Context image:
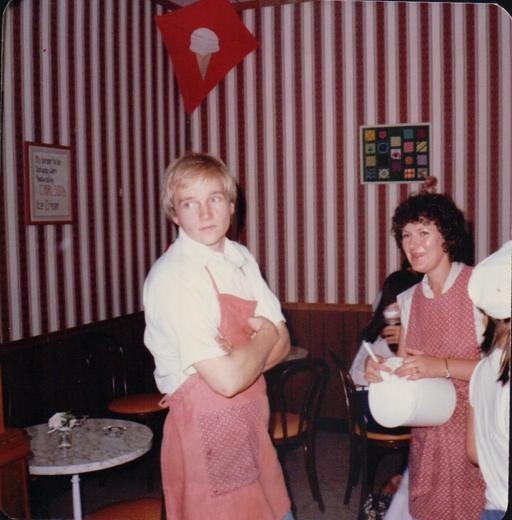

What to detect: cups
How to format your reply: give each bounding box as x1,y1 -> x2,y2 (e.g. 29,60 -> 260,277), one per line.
383,310 -> 401,352
55,431 -> 72,450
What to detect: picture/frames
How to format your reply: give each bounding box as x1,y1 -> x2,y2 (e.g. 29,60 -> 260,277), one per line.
358,122 -> 432,186
23,140 -> 77,227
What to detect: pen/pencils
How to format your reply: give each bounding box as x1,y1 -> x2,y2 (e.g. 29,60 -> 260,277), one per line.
361,340 -> 380,363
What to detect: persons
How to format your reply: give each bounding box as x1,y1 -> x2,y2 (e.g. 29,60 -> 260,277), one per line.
142,151 -> 294,519
347,194 -> 511,520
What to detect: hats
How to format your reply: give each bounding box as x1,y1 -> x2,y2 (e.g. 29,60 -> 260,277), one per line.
467,239 -> 512,320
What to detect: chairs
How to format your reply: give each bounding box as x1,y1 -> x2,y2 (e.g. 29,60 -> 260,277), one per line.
269,361 -> 412,518
84,348 -> 165,427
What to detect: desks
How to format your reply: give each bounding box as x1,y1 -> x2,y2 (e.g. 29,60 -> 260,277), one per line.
19,418 -> 156,520
